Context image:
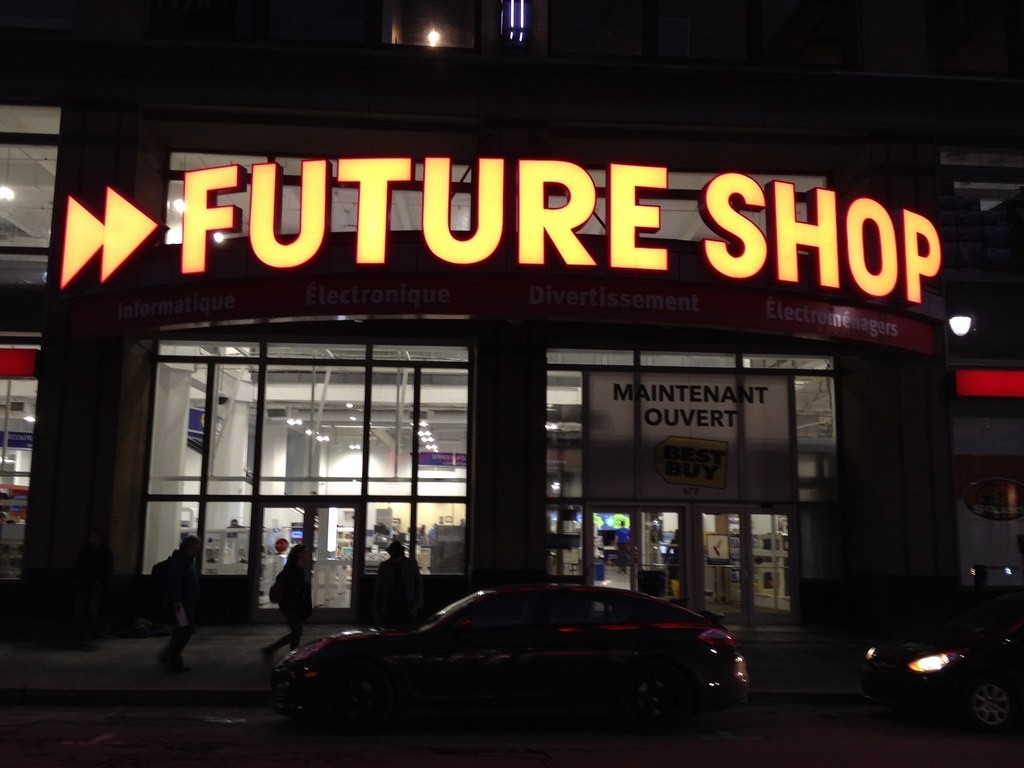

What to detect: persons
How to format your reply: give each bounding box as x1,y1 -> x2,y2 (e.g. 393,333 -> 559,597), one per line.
615,519 -> 630,573
161,536 -> 200,663
378,540 -> 424,626
71,528 -> 115,640
260,545 -> 310,657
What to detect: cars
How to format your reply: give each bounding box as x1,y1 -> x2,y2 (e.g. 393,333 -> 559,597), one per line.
270,584 -> 750,739
858,589 -> 1024,736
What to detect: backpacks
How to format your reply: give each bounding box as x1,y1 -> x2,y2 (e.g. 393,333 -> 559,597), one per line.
146,560 -> 178,603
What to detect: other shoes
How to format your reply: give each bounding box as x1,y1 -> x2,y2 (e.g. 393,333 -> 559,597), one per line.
74,632 -> 91,641
162,654 -> 183,669
262,648 -> 275,665
